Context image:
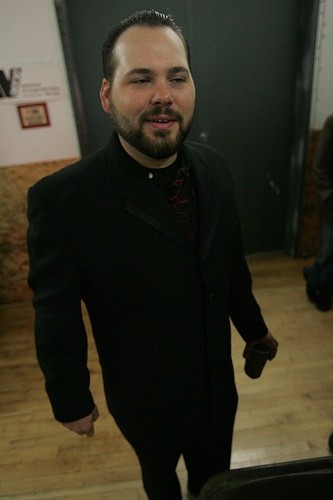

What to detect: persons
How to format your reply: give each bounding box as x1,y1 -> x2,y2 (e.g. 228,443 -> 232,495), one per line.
27,9 -> 279,498
